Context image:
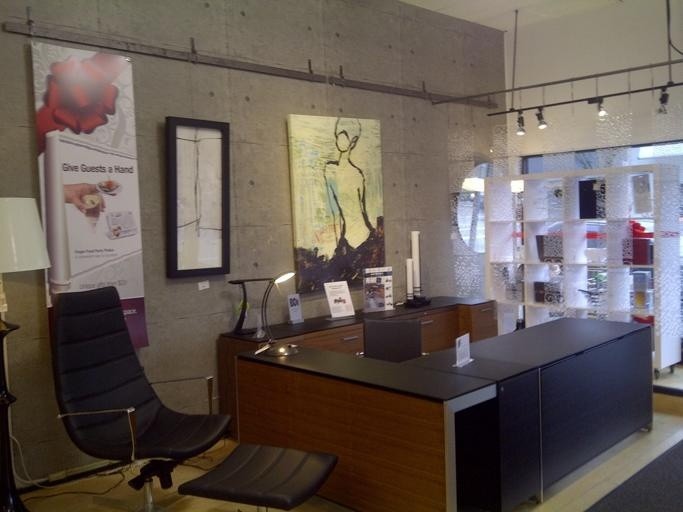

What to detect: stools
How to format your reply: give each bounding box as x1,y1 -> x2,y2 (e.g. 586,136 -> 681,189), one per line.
179,441 -> 339,512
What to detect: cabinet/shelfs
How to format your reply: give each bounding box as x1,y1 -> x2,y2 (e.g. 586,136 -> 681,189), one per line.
483,164 -> 681,378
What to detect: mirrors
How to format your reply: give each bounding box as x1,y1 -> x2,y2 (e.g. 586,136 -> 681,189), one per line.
457,162 -> 486,254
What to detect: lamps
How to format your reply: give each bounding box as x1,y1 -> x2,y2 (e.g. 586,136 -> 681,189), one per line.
596,100 -> 607,117
656,88 -> 670,115
535,107 -> 549,131
0,196 -> 53,493
260,271 -> 299,356
514,110 -> 528,137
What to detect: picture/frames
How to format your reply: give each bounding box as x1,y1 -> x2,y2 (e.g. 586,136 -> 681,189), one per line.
164,115 -> 231,278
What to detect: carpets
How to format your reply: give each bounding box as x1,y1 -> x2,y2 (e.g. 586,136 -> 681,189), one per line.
584,438 -> 682,511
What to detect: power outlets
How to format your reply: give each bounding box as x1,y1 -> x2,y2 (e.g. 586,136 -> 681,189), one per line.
197,280 -> 210,292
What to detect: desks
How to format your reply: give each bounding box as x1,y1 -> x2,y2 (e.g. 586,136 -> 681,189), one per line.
217,296 -> 499,421
237,341 -> 497,512
411,317 -> 655,512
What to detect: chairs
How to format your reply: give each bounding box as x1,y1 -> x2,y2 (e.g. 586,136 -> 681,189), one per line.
50,286 -> 232,512
